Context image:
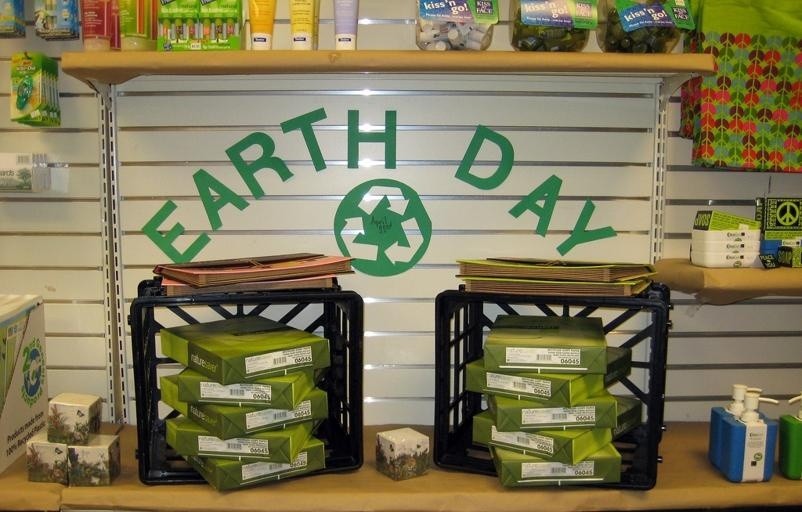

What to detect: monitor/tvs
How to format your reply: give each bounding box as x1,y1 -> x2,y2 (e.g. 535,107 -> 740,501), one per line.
457,256 -> 656,296
153,253 -> 355,297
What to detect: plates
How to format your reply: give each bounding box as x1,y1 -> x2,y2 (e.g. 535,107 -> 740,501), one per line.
57,48 -> 798,512
